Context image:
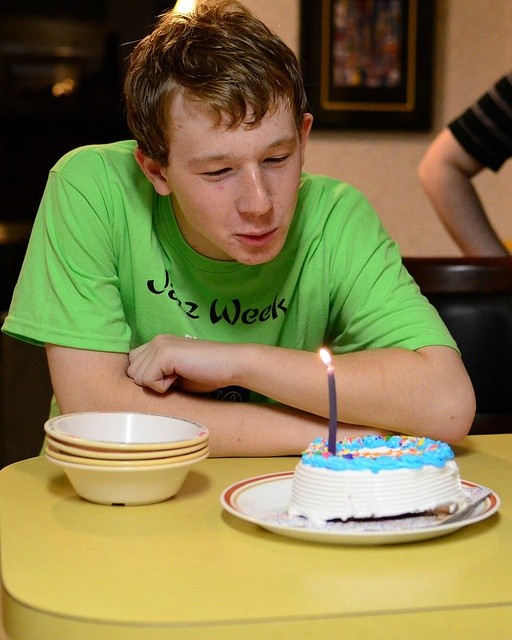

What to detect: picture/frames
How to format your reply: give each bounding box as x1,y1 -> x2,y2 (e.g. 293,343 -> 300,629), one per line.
296,2 -> 434,133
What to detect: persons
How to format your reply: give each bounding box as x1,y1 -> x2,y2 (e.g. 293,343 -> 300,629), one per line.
3,2 -> 479,458
418,71 -> 512,259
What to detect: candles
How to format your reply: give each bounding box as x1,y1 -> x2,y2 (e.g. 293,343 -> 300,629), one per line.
318,346 -> 340,456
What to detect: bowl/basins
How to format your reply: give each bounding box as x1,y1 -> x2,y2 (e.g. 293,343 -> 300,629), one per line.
44,410 -> 210,507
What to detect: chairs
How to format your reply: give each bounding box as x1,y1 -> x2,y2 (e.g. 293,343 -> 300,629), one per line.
396,257 -> 509,432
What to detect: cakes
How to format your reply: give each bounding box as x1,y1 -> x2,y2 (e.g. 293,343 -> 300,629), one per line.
288,437 -> 461,521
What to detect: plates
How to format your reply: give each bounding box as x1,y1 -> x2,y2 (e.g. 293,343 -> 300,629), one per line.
222,471 -> 501,547
